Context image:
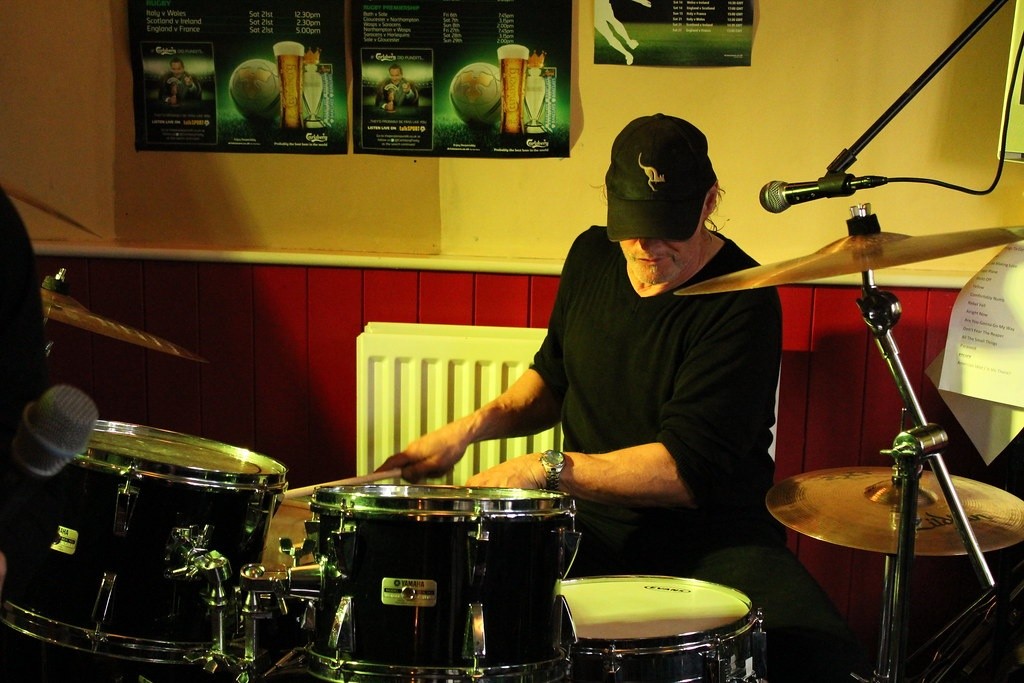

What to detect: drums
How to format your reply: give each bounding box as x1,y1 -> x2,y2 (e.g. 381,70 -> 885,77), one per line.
248,566 -> 322,683
0,417 -> 288,682
305,484 -> 579,683
556,574 -> 769,683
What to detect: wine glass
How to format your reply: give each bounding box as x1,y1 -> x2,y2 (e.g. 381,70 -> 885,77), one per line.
299,63 -> 326,129
522,67 -> 546,134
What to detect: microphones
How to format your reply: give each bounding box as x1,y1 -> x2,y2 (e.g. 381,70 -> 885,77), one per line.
759,176 -> 888,214
0,384 -> 100,553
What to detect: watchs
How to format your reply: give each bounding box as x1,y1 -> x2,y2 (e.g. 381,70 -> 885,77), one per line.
538,449 -> 566,491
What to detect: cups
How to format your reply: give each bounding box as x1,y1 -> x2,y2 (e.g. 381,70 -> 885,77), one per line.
271,40 -> 306,133
497,43 -> 529,135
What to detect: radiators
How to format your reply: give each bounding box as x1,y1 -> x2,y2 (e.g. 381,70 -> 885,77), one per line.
356,322 -> 564,485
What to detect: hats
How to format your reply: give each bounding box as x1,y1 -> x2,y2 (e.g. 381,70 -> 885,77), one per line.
605,112 -> 718,242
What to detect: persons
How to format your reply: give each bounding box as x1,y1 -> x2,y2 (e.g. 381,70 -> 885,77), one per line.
375,113 -> 863,683
157,57 -> 202,110
376,64 -> 419,112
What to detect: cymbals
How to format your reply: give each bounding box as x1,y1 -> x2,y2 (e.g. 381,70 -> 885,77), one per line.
672,200 -> 1024,293
765,466 -> 1024,557
34,268 -> 212,365
